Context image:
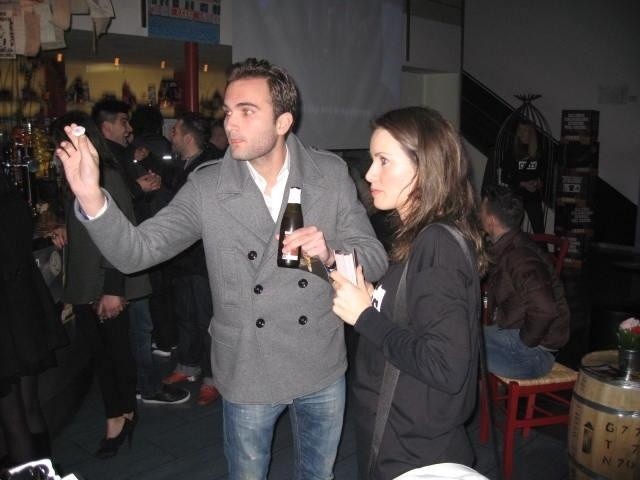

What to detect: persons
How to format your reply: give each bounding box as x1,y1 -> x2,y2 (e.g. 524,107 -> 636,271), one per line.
501,121 -> 570,254
0,172 -> 72,480
479,185 -> 571,380
55,57 -> 389,480
330,107 -> 493,480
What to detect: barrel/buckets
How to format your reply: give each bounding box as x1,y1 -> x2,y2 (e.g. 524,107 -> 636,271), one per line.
566,350 -> 640,480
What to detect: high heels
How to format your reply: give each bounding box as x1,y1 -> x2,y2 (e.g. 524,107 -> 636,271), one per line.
94,412 -> 138,458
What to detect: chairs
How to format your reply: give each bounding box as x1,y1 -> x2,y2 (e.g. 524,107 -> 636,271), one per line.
478,233 -> 581,480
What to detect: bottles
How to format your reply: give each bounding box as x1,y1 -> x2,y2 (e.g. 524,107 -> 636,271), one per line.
277,187 -> 305,269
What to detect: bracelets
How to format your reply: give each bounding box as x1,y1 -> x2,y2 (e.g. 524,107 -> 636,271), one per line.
327,263 -> 337,270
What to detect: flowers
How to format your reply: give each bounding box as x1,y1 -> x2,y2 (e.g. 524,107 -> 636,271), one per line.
612,315 -> 640,353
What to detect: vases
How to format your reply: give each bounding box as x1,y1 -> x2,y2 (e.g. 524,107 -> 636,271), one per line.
612,344 -> 640,390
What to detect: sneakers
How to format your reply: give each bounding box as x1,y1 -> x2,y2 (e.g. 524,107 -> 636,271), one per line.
160,370 -> 185,384
142,383 -> 190,404
136,390 -> 142,400
198,386 -> 219,406
